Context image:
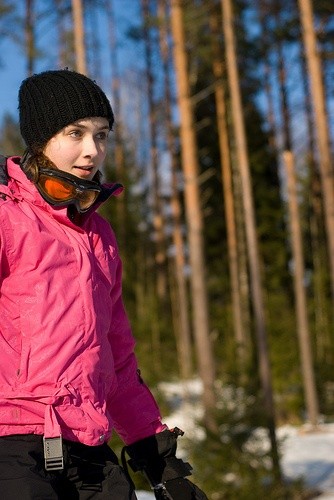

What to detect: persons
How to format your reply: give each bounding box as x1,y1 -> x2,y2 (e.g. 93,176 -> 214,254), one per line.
1,70 -> 210,500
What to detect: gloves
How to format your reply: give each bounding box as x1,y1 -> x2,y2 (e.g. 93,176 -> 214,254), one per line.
127,423 -> 212,500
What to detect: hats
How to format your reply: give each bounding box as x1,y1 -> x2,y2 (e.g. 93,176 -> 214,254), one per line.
17,68 -> 116,149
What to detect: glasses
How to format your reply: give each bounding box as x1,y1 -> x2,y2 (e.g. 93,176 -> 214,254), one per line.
23,154 -> 102,214
